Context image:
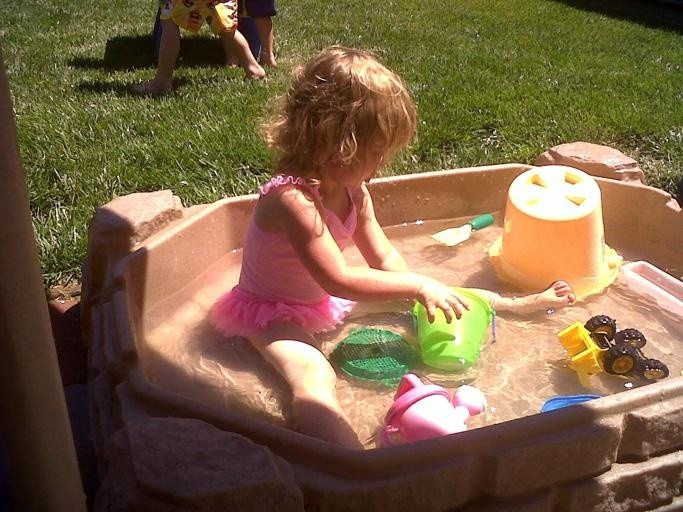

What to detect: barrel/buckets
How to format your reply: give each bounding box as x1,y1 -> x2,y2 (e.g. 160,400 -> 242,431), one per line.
412,285 -> 497,372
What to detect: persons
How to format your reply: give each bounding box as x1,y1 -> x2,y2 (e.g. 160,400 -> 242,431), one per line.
223,1 -> 278,71
209,46 -> 576,452
132,0 -> 265,96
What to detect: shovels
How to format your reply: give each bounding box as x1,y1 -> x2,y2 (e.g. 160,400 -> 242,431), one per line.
430,214 -> 494,246
